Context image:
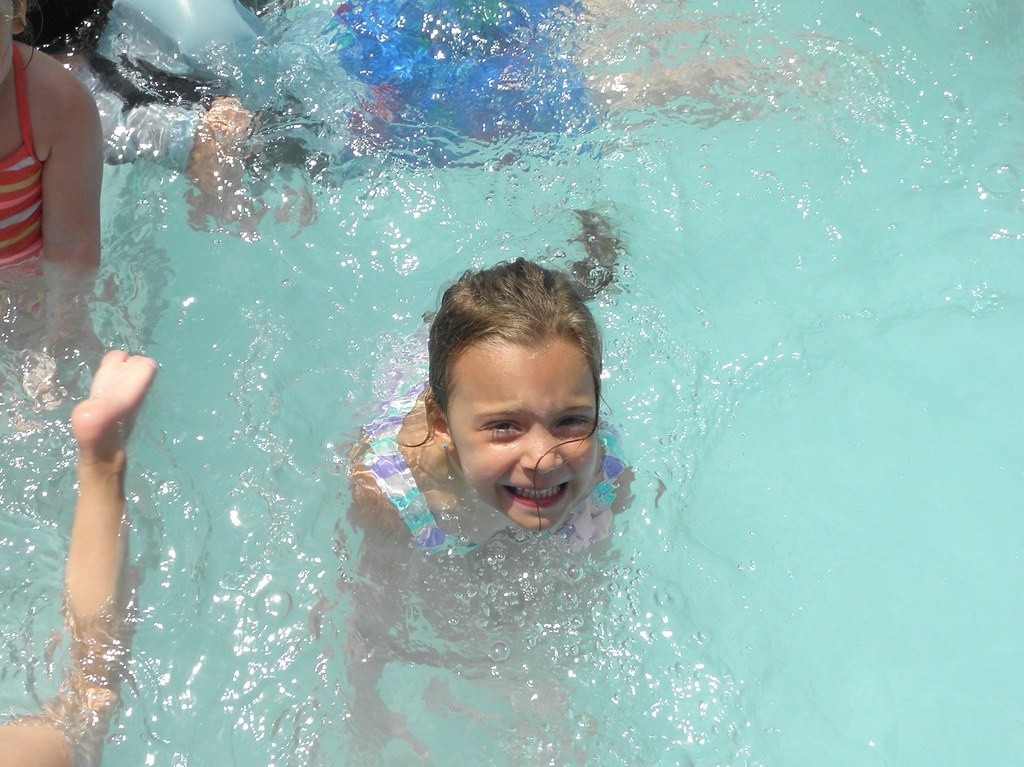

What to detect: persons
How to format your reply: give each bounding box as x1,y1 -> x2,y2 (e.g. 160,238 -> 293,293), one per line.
0,0 -> 103,271
338,261 -> 634,560
0,349 -> 158,765
